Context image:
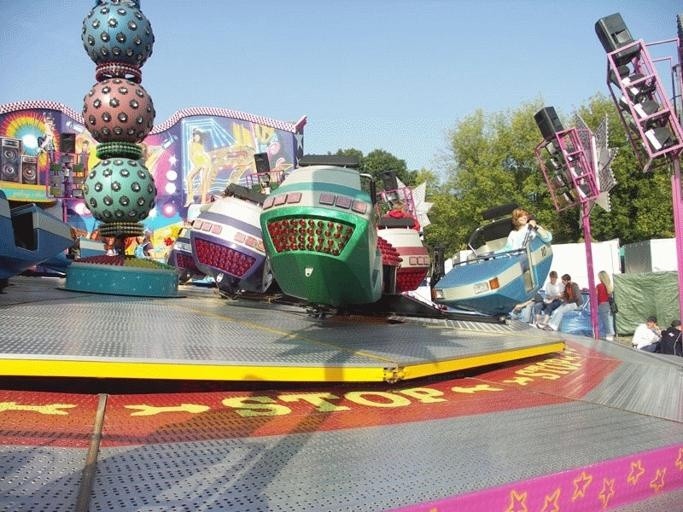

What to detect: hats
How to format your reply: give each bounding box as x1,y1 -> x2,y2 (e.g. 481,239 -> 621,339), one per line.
647,316 -> 657,322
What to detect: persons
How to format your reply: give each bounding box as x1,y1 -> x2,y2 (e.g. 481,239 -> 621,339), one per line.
511,293 -> 544,323
659,320 -> 683,357
631,316 -> 662,353
482,207 -> 553,256
182,129 -> 210,208
535,274 -> 583,331
79,141 -> 91,177
529,270 -> 563,328
583,270 -> 615,341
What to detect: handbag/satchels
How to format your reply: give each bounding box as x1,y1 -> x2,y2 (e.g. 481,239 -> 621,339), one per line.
608,296 -> 618,313
534,293 -> 561,309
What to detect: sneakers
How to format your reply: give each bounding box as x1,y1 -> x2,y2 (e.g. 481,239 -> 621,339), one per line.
520,318 -> 558,331
606,336 -> 613,341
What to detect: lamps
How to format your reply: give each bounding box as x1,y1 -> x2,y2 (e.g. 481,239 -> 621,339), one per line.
607,64 -> 673,155
534,139 -> 591,205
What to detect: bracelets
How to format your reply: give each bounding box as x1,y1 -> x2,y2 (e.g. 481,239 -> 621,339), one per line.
534,224 -> 541,230
